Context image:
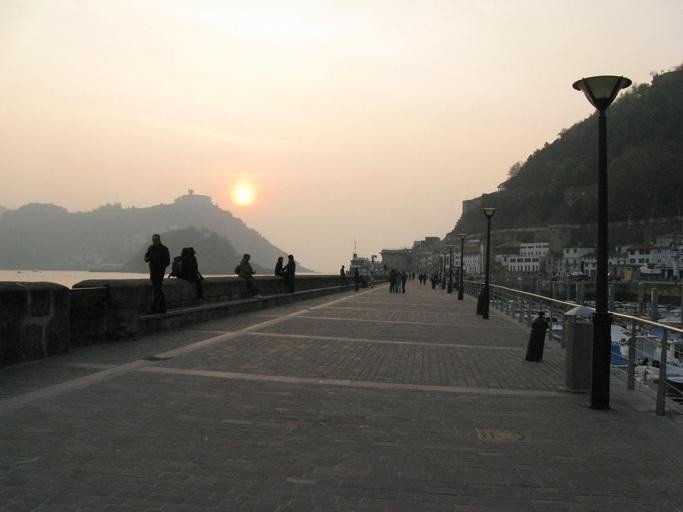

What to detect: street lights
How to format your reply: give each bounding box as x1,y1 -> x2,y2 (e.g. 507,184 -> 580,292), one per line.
436,246 -> 454,294
479,206 -> 496,320
457,233 -> 467,300
571,75 -> 633,406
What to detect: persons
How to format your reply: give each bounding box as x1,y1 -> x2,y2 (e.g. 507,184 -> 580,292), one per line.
275,257 -> 288,277
283,254 -> 296,293
340,265 -> 350,289
387,268 -> 438,293
145,234 -> 208,315
352,267 -> 361,291
238,254 -> 266,299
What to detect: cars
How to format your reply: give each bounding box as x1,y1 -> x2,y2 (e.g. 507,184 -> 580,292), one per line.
566,271 -> 588,280
607,272 -> 623,280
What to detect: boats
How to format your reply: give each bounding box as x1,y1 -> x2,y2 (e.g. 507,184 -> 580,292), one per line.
345,242 -> 384,276
492,296 -> 682,407
87,263 -> 127,272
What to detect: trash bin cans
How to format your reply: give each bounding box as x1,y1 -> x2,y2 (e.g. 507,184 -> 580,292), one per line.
566,305 -> 594,393
